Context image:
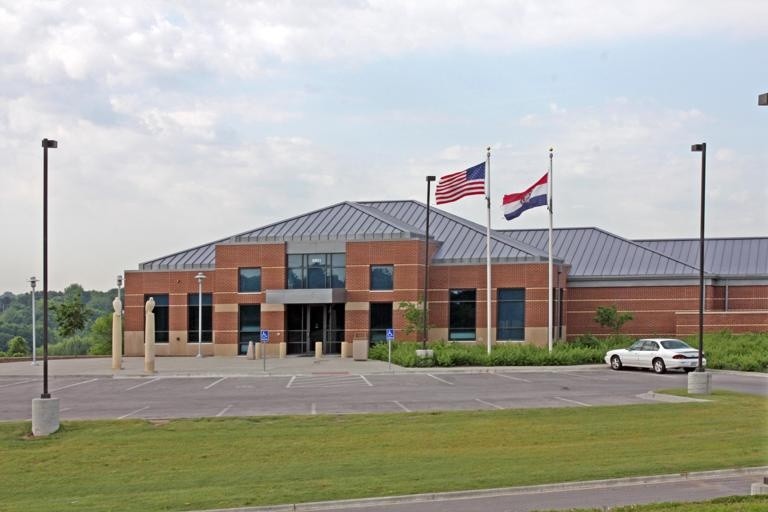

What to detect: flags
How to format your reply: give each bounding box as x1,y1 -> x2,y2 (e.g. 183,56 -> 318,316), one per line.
434,160 -> 487,204
502,172 -> 549,221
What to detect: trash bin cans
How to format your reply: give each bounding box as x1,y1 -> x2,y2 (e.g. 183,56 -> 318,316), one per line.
255,342 -> 261,359
315,342 -> 322,358
280,342 -> 286,359
341,342 -> 348,358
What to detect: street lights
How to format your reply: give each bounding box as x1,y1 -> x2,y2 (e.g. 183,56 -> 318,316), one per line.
690,142 -> 707,372
423,174 -> 436,349
193,270 -> 208,358
26,134 -> 59,397
113,273 -> 127,362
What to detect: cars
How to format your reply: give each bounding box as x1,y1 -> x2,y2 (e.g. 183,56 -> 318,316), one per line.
604,337 -> 707,374
